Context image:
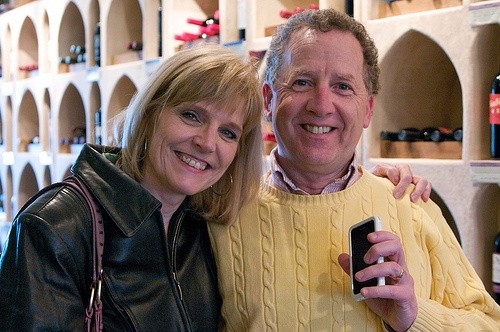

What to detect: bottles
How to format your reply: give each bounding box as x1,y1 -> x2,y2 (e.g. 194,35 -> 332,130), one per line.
491,231 -> 500,304
173,10 -> 219,43
279,4 -> 319,20
489,76 -> 500,158
378,125 -> 464,143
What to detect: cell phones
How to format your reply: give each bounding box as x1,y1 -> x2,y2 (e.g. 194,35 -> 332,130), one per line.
348,215 -> 386,302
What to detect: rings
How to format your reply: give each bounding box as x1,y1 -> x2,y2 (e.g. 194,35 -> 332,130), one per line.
393,267 -> 404,279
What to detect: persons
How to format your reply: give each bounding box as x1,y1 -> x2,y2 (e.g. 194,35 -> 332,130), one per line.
200,10 -> 500,332
0,45 -> 433,332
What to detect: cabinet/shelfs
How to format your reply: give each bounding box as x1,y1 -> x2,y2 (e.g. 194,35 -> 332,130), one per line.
0,0 -> 499,303
